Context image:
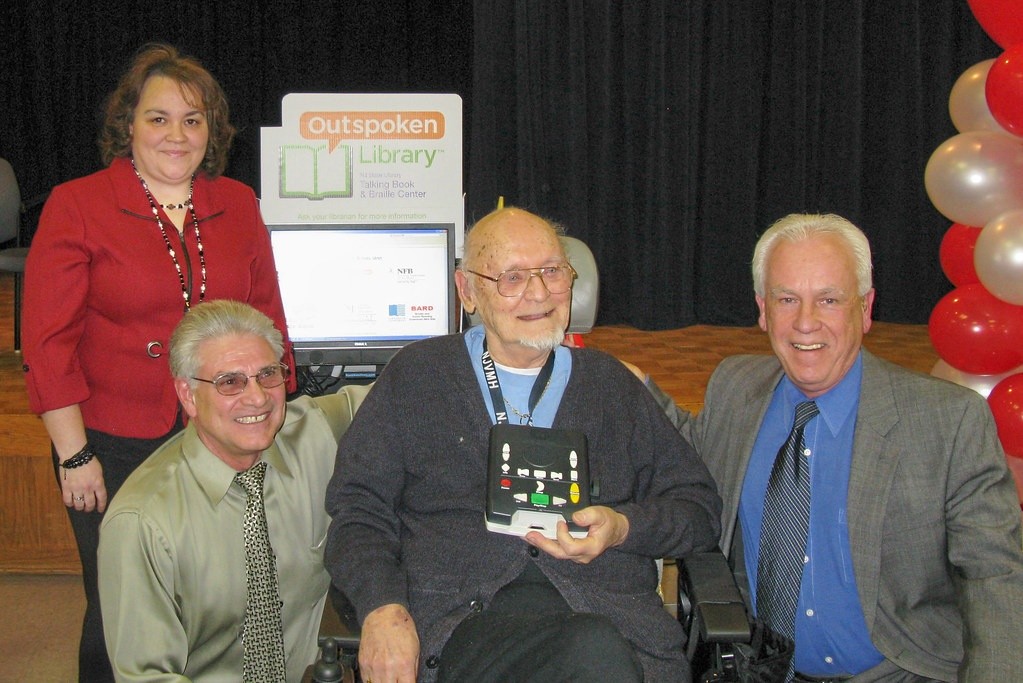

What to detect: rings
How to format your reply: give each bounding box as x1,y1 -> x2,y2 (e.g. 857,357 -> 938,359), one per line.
73,497 -> 84,501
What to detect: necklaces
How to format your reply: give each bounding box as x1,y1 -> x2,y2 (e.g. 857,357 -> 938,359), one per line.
155,201 -> 190,210
129,158 -> 206,312
502,380 -> 550,419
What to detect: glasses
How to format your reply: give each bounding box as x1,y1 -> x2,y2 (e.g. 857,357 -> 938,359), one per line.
466,259 -> 578,298
186,361 -> 289,397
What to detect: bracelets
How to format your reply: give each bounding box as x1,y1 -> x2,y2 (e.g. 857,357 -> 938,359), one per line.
57,442 -> 95,468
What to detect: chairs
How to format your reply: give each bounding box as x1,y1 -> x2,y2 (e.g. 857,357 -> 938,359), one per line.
0,156 -> 30,354
302,544 -> 754,683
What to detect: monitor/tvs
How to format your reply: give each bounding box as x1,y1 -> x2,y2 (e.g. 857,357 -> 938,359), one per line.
266,224 -> 456,379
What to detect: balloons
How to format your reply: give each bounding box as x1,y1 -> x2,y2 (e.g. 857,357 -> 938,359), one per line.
925,0 -> 1023,513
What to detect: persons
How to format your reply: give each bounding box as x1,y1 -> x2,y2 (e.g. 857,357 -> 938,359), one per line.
623,213 -> 1023,681
323,209 -> 724,682
22,44 -> 297,683
97,298 -> 374,683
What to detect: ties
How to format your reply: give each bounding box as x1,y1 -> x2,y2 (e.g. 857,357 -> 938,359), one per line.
755,401 -> 820,681
232,462 -> 286,683
